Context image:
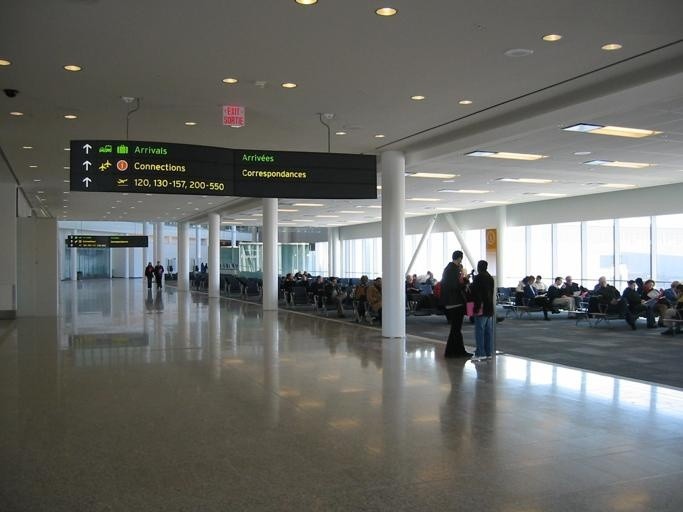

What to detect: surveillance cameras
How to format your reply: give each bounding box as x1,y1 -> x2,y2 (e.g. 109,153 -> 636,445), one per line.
3,89 -> 18,98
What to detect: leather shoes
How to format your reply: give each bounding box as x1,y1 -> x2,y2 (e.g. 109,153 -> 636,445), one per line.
647,324 -> 657,328
658,323 -> 666,327
631,324 -> 637,329
661,329 -> 673,335
551,308 -> 560,313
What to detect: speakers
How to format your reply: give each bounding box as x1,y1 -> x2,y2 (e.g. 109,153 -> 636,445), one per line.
309,242 -> 315,251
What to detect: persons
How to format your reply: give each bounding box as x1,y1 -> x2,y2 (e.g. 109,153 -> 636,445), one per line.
442,251 -> 474,358
154,261 -> 164,288
193,262 -> 207,273
279,270 -> 442,323
454,263 -> 470,293
515,273 -> 682,338
144,262 -> 154,289
470,359 -> 496,451
144,289 -> 153,314
469,260 -> 495,360
437,358 -> 467,451
154,287 -> 164,314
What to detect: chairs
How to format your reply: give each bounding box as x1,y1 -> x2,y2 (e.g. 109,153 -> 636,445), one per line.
190,272 -> 683,335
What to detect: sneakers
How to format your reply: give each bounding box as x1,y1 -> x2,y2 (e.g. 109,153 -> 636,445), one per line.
445,352 -> 492,361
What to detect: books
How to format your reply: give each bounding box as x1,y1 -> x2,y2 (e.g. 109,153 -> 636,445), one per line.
465,301 -> 483,317
